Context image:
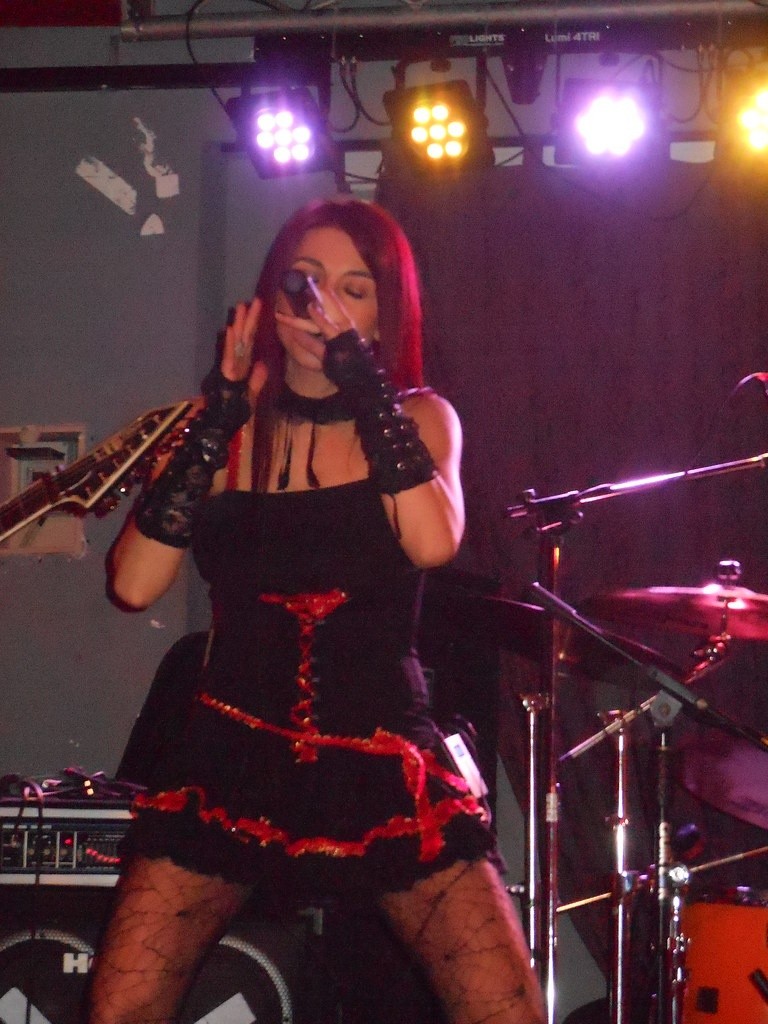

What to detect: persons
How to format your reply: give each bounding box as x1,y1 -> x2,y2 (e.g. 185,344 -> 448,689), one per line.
89,199 -> 552,1024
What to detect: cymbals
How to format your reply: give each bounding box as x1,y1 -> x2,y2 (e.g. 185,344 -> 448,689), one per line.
477,594 -> 683,690
571,583 -> 768,639
670,727 -> 768,830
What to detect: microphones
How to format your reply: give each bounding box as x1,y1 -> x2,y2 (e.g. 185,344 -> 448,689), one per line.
282,270 -> 318,320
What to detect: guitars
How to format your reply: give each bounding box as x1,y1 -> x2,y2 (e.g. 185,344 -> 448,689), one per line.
0,397 -> 207,541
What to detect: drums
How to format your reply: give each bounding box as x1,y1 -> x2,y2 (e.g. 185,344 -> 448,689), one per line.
668,881 -> 768,1023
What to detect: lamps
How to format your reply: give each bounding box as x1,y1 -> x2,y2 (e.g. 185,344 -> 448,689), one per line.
550,44 -> 672,167
224,31 -> 342,181
383,45 -> 496,179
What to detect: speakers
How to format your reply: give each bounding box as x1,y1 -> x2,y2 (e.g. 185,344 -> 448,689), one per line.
0,897 -> 343,1024
